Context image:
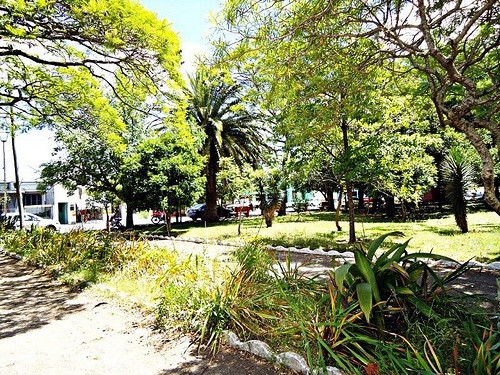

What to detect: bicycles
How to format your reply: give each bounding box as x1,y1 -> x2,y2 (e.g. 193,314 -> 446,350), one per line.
108,203 -> 182,229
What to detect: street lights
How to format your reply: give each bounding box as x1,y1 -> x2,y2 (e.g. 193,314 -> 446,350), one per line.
0,123 -> 14,226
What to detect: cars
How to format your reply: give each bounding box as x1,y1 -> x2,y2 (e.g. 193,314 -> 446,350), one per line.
187,203 -> 232,221
3,212 -> 61,234
228,181 -> 483,223
